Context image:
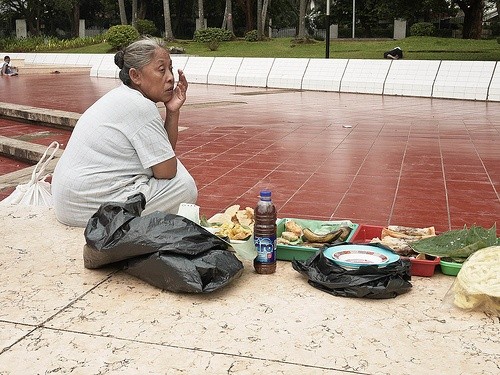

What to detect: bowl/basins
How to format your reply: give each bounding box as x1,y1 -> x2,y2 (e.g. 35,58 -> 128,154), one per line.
323,245 -> 399,268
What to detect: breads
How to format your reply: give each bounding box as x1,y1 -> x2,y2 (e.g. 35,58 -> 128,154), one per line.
285,220 -> 303,238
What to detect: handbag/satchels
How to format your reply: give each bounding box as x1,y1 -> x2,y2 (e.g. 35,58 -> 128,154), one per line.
0,141 -> 59,207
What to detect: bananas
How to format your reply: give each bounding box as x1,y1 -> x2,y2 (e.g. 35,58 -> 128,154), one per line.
302,228 -> 343,242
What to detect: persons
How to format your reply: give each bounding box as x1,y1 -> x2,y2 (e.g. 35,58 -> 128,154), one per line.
0,55 -> 19,78
52,34 -> 198,228
384,46 -> 403,60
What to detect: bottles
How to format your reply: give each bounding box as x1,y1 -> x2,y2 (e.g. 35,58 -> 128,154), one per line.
254,191 -> 278,275
